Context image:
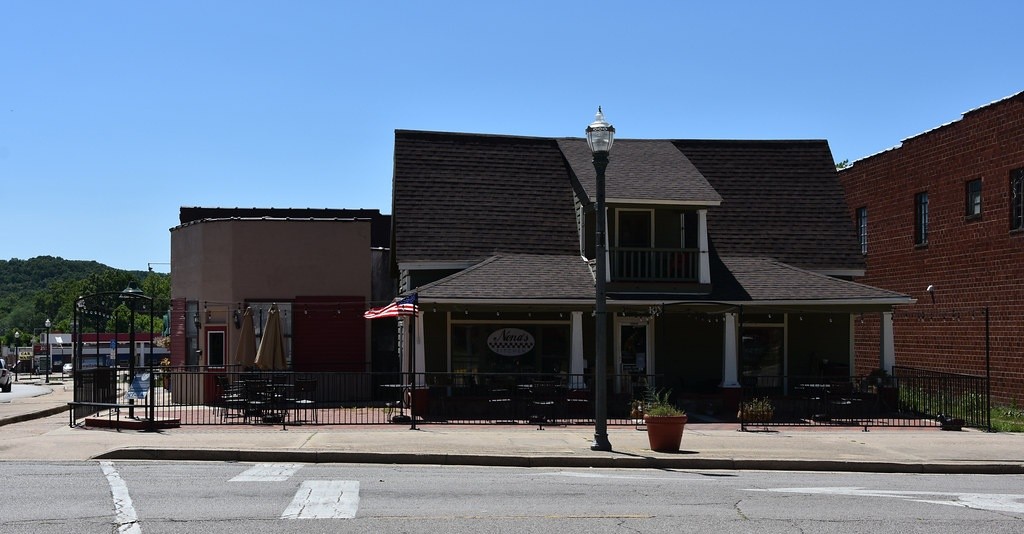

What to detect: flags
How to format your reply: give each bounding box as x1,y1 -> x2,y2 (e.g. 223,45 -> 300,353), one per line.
363,292 -> 419,320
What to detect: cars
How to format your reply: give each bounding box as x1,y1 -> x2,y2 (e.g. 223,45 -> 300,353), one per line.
63,363 -> 73,373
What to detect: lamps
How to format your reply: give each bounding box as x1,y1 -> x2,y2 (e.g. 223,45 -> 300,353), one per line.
194,312 -> 201,329
233,311 -> 240,330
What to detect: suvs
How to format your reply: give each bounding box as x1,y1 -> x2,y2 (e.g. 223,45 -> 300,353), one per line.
0,359 -> 13,392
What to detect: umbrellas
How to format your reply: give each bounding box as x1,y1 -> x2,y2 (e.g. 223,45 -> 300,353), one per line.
253,302 -> 287,416
235,306 -> 260,371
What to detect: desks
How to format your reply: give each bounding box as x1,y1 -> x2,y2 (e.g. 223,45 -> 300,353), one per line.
268,384 -> 295,388
233,380 -> 273,383
380,383 -> 425,423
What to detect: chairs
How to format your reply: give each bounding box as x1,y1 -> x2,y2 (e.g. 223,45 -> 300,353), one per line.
218,370 -> 318,424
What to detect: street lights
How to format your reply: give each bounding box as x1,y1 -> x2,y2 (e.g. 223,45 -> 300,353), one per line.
78,296 -> 85,388
45,319 -> 50,382
584,105 -> 615,451
14,331 -> 19,381
119,280 -> 143,420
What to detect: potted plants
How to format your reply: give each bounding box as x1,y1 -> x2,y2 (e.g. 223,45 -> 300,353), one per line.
738,397 -> 773,421
640,375 -> 688,453
